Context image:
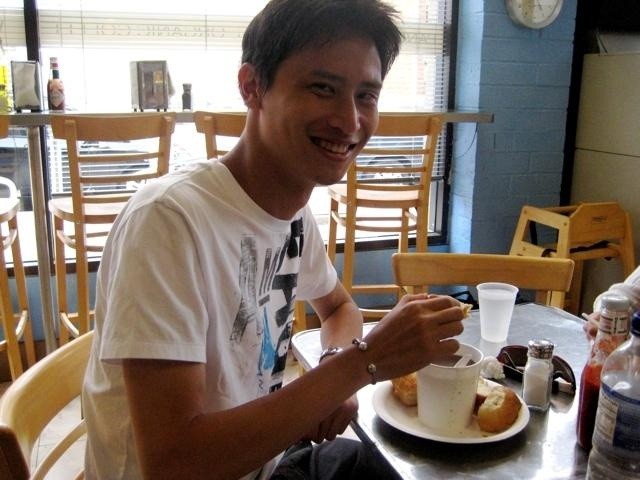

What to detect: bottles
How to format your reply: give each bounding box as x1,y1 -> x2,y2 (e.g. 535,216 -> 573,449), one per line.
586,313 -> 640,480
576,293 -> 632,453
182,83 -> 192,111
521,338 -> 556,415
0,84 -> 9,115
47,57 -> 65,113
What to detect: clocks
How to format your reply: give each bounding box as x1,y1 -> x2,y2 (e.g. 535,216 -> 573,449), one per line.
507,0 -> 565,32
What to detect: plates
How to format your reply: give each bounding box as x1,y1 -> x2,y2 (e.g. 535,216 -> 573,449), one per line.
372,378 -> 531,444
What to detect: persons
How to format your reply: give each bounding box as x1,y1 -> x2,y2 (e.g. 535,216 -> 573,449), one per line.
584,263 -> 640,342
78,0 -> 410,480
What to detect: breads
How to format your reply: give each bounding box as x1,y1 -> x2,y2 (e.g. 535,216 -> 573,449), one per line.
474,380 -> 488,410
392,372 -> 417,407
477,386 -> 522,432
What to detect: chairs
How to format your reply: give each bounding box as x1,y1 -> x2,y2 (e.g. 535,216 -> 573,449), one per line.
506,196 -> 635,311
2,328 -> 102,480
191,109 -> 249,160
389,249 -> 574,317
326,114 -> 445,318
0,111 -> 38,385
44,111 -> 177,352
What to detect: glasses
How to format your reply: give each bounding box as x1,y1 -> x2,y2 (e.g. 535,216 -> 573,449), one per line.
495,346 -> 575,396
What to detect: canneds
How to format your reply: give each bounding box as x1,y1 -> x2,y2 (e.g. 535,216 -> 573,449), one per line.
521,338 -> 554,411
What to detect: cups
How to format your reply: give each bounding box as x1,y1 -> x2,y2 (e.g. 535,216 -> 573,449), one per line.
476,281 -> 519,344
416,343 -> 484,432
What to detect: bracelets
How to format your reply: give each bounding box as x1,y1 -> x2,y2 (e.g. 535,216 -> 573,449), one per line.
318,346 -> 345,362
352,337 -> 377,386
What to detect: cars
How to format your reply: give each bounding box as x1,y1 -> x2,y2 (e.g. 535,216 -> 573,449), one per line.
340,138 -> 431,182
1,124 -> 152,203
